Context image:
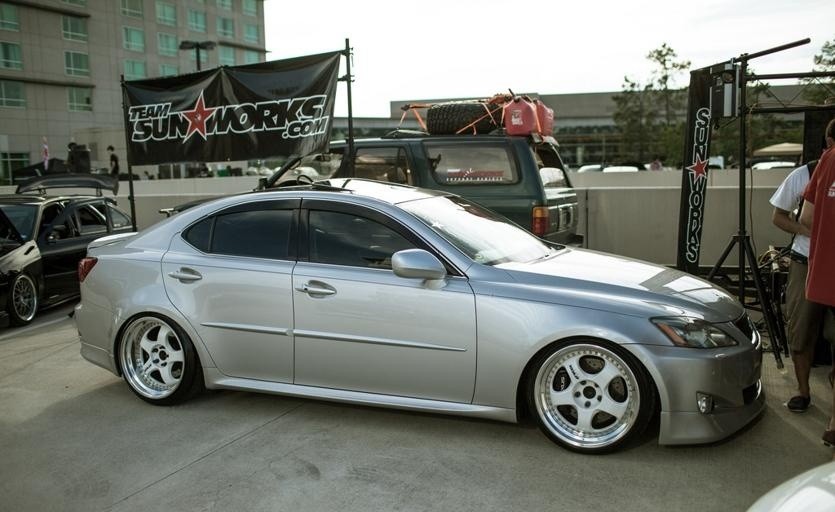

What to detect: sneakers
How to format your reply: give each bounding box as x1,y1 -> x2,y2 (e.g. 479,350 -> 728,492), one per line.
788,395 -> 811,412
822,429 -> 835,446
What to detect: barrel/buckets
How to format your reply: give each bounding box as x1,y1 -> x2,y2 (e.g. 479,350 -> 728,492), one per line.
504,93 -> 553,136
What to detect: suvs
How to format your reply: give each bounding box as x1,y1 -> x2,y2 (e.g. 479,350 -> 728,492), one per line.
158,129 -> 584,271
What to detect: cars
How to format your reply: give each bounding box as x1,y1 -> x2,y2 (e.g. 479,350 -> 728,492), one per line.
0,172 -> 134,331
71,152 -> 767,456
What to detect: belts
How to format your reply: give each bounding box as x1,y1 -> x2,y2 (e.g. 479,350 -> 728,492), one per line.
789,250 -> 808,265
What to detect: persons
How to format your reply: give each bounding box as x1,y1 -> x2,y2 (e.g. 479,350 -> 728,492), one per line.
648,154 -> 664,170
105,144 -> 120,182
796,143 -> 834,448
767,118 -> 835,413
